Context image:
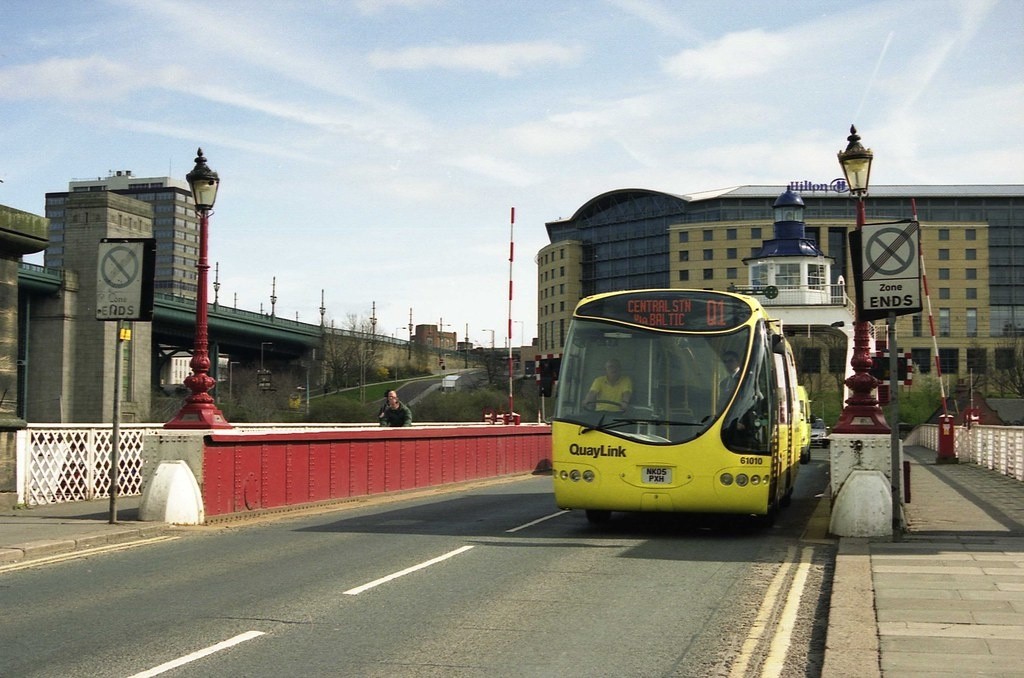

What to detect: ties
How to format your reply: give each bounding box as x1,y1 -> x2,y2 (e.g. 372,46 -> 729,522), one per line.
727,375 -> 733,390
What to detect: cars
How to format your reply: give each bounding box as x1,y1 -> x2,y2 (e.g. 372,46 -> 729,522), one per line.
810,417 -> 831,448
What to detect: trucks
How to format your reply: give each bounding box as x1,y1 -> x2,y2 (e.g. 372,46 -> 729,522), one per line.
442,375 -> 462,396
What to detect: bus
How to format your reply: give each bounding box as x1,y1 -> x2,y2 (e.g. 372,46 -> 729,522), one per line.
536,289 -> 801,528
797,384 -> 811,465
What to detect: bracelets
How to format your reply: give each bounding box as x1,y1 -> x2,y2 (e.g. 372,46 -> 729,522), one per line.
622,400 -> 629,406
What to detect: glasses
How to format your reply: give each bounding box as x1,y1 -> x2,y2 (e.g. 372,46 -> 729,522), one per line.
723,359 -> 732,363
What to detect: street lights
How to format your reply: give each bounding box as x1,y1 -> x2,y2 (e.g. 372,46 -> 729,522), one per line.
444,353 -> 452,377
514,320 -> 523,345
836,121 -> 893,437
482,328 -> 495,348
395,327 -> 406,337
260,340 -> 274,369
360,348 -> 374,407
229,361 -> 240,402
161,144 -> 237,430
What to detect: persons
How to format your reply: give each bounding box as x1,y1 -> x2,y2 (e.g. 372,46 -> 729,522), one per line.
379,397 -> 412,427
378,390 -> 398,422
582,358 -> 632,411
718,351 -> 755,428
